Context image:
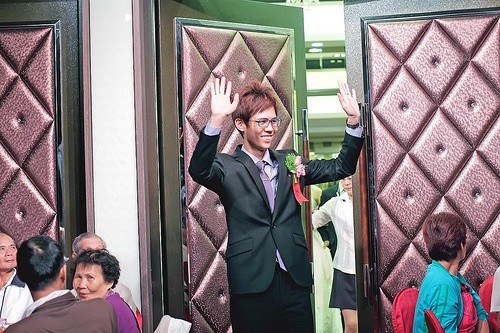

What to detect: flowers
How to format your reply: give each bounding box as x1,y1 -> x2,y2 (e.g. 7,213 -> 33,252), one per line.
284,152 -> 306,184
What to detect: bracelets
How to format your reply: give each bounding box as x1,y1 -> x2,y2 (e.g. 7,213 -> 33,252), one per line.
345,118 -> 359,129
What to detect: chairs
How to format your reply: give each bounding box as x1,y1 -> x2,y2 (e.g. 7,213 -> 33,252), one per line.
393,276 -> 500,333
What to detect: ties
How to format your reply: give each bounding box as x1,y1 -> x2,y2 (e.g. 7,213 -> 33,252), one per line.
256,161 -> 288,272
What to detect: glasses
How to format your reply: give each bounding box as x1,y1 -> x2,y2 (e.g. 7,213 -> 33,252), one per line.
248,117 -> 281,126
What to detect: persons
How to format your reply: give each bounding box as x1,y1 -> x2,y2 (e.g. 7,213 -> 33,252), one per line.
310,185 -> 322,212
310,175 -> 358,333
490,265 -> 500,324
318,181 -> 346,333
0,232 -> 34,333
72,249 -> 142,333
188,77 -> 364,333
70,232 -> 136,315
0,236 -> 118,333
412,211 -> 489,333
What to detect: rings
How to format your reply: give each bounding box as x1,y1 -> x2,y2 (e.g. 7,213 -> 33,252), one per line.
346,92 -> 349,95
